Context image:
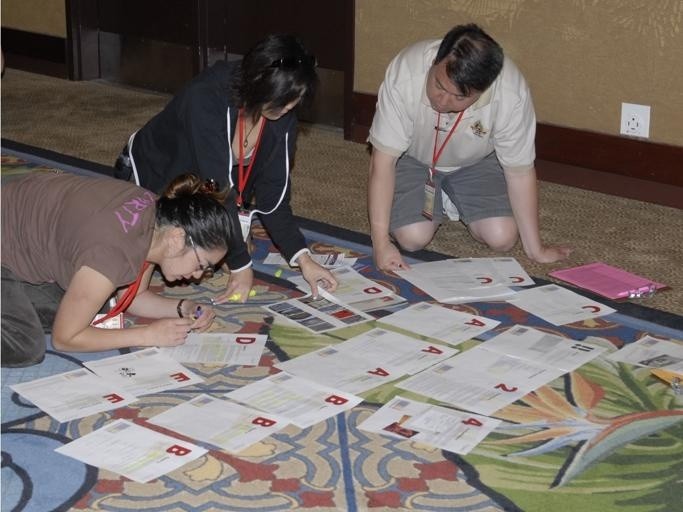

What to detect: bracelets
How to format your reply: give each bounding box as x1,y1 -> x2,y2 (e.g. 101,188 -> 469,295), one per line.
176,299 -> 185,318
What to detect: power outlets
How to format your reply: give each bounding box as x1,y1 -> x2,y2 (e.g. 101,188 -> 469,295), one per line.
620,102 -> 651,138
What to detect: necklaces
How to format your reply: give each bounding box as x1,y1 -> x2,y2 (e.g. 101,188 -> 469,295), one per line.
243,115 -> 260,148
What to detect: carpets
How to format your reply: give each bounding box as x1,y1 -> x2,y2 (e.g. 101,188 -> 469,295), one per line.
1,136 -> 683,512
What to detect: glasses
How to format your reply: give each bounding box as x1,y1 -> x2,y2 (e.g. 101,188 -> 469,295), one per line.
189,236 -> 214,280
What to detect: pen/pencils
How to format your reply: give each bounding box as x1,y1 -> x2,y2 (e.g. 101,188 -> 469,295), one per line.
392,262 -> 402,268
391,263 -> 400,267
193,306 -> 201,321
227,290 -> 256,302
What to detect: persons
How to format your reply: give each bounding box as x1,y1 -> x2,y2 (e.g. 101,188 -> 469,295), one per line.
0,165 -> 236,371
365,19 -> 579,274
112,30 -> 339,307
